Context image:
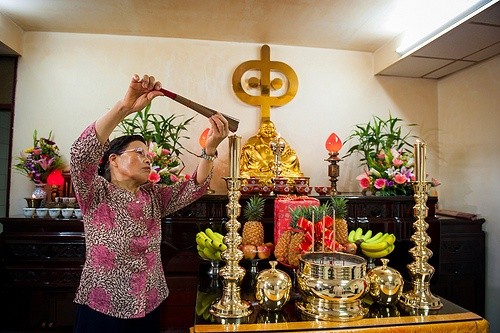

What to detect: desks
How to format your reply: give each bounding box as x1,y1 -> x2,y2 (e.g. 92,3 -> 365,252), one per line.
163,196 -> 437,333
190,275 -> 491,333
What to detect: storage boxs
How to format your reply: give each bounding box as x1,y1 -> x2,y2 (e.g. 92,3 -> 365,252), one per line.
273,196 -> 320,266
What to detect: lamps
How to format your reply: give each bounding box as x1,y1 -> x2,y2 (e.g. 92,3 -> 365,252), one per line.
395,0 -> 490,56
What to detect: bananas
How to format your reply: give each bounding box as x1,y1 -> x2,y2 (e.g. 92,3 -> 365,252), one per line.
196,292 -> 222,320
348,227 -> 396,258
196,228 -> 227,259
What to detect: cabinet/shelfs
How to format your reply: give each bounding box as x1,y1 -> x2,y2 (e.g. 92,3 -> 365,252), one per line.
435,214 -> 486,316
0,217 -> 84,333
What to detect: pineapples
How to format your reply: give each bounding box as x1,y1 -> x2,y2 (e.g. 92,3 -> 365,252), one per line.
241,196 -> 266,246
273,196 -> 348,265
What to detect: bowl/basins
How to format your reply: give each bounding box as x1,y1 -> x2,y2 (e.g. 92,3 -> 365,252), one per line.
239,179 -> 332,195
74,209 -> 83,219
61,209 -> 74,219
22,208 -> 35,218
49,208 -> 61,218
36,209 -> 50,218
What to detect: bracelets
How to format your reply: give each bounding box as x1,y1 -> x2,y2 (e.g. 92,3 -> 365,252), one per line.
201,149 -> 218,162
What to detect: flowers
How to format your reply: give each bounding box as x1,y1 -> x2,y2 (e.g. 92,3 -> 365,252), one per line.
11,130 -> 64,180
122,104 -> 184,183
345,116 -> 412,195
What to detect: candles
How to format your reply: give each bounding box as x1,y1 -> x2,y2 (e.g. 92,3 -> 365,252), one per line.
228,134 -> 240,178
415,141 -> 426,183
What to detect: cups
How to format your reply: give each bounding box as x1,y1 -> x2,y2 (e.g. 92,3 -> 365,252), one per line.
54,196 -> 76,203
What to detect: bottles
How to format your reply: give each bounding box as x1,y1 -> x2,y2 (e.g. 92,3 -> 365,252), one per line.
253,260 -> 291,310
368,258 -> 404,306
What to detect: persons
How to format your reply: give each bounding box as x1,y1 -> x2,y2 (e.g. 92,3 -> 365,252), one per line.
240,120 -> 303,178
70,74 -> 229,333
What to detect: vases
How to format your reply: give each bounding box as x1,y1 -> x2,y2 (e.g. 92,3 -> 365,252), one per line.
32,184 -> 47,199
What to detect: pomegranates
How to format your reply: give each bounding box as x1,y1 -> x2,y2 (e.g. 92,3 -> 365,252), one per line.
242,243 -> 270,259
344,242 -> 357,255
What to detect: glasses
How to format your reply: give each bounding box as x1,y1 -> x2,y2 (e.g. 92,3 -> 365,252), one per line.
120,147 -> 153,159
